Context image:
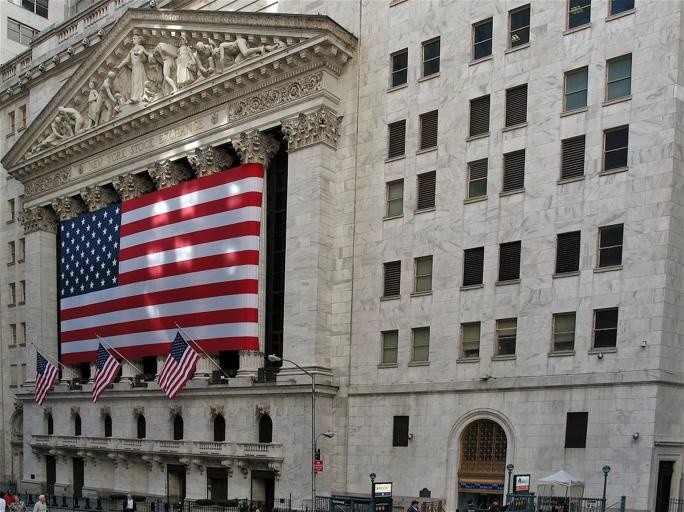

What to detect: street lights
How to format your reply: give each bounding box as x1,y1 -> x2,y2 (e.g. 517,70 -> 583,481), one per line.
507,464 -> 514,494
315,431 -> 335,440
600,466 -> 611,512
370,473 -> 376,512
267,355 -> 316,511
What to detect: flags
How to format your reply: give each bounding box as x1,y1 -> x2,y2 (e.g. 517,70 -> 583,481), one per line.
34,351 -> 60,406
60,162 -> 264,365
156,332 -> 201,400
90,341 -> 121,403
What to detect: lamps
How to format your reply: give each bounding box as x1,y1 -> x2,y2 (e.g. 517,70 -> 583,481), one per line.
633,432 -> 638,439
597,352 -> 603,359
641,341 -> 646,347
408,434 -> 413,439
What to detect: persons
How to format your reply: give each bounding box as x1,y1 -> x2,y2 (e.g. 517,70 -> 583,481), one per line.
4,488 -> 15,507
29,34 -> 277,154
122,493 -> 137,512
407,501 -> 420,512
32,495 -> 48,512
10,496 -> 27,512
255,507 -> 263,512
0,491 -> 9,512
489,498 -> 509,512
464,500 -> 480,512
